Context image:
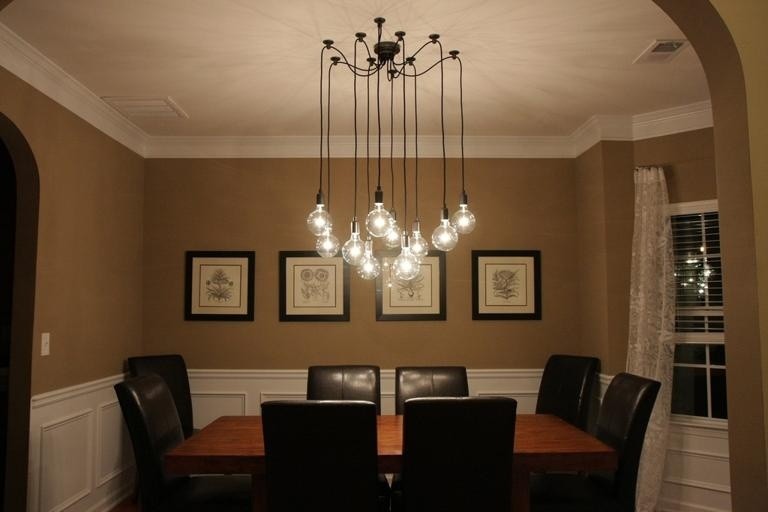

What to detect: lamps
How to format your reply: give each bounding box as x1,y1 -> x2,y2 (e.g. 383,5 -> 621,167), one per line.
309,15 -> 475,284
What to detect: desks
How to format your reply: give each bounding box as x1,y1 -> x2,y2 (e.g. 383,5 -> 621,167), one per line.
175,410 -> 613,510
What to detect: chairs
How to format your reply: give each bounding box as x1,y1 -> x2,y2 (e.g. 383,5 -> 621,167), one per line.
395,365 -> 470,415
535,354 -> 601,430
118,380 -> 249,506
259,396 -> 393,510
128,349 -> 202,439
400,392 -> 515,511
538,372 -> 663,509
306,364 -> 385,412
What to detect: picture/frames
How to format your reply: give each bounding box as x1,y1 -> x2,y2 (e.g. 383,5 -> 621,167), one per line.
182,249 -> 254,321
470,249 -> 543,321
276,247 -> 350,320
373,248 -> 447,321
